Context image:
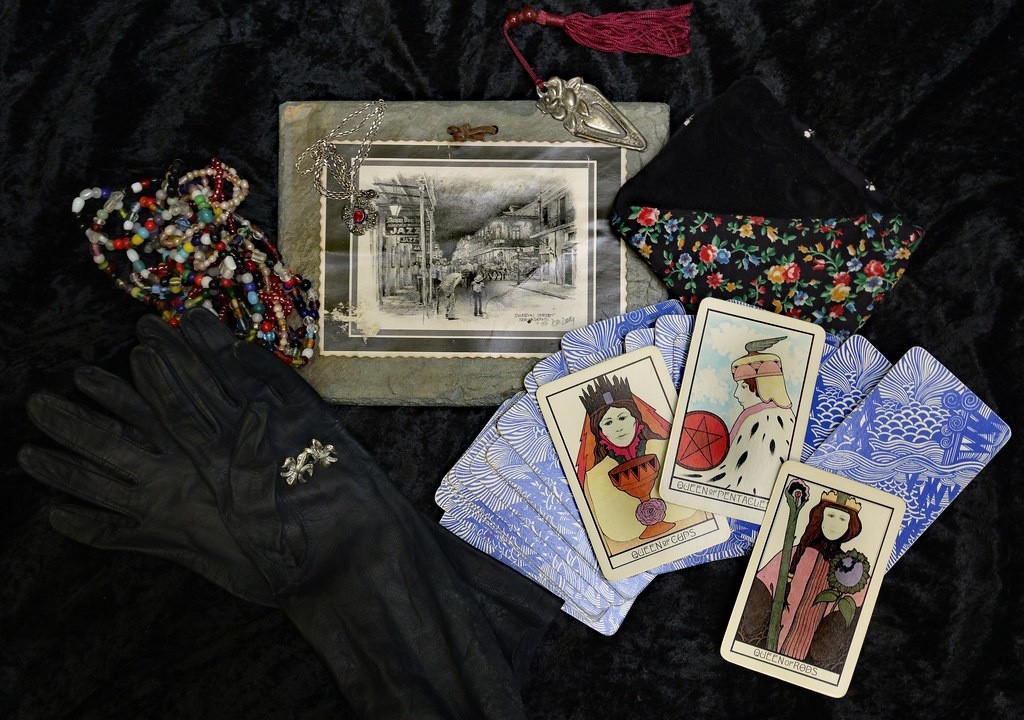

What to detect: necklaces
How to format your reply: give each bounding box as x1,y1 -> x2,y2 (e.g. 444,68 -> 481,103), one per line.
293,101 -> 388,237
69,155 -> 320,368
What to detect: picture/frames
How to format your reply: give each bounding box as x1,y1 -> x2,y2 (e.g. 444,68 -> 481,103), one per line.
277,101 -> 670,407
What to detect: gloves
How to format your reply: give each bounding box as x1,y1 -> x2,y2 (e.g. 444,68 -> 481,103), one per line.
132,307 -> 524,720
17,363 -> 565,693
